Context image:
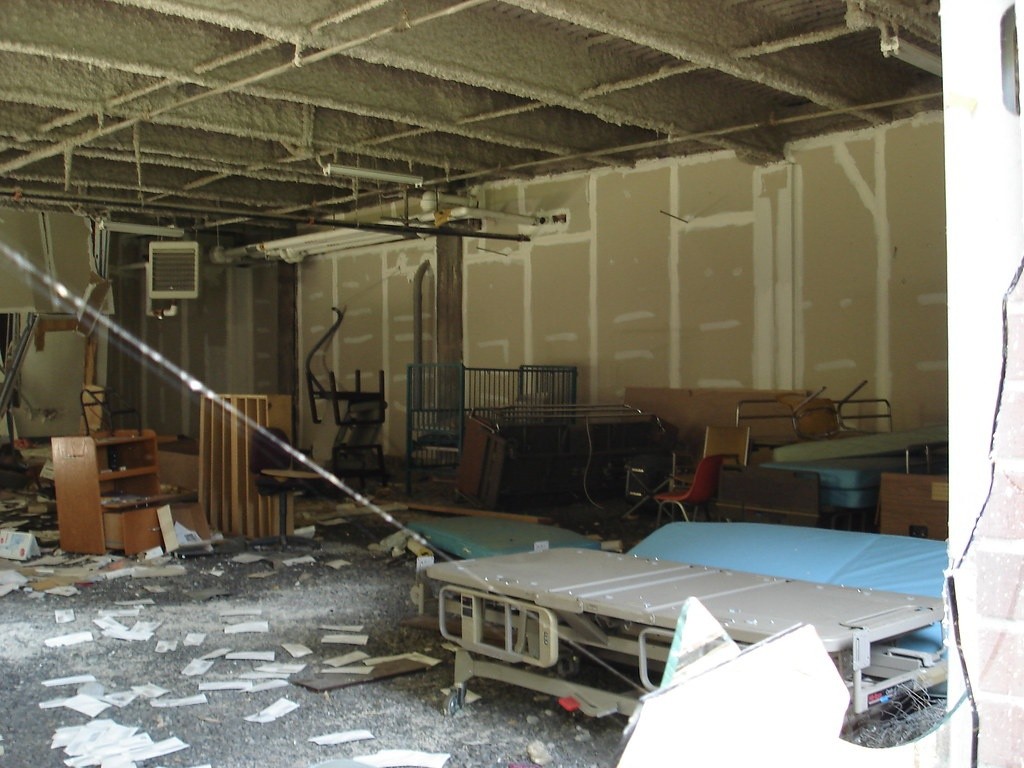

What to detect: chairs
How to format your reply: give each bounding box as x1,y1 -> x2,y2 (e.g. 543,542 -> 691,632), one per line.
623,376 -> 868,530
246,429 -> 324,551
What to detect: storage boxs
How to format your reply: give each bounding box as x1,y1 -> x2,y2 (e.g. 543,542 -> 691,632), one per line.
156,502 -> 211,553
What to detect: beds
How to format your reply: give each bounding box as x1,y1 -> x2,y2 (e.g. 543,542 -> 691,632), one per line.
405,363 -> 578,500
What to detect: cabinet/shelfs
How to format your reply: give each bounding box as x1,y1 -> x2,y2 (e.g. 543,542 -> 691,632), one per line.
50,428 -> 179,556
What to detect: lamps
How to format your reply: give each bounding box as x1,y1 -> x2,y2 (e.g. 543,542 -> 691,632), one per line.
321,164 -> 423,188
98,216 -> 185,237
880,37 -> 942,78
